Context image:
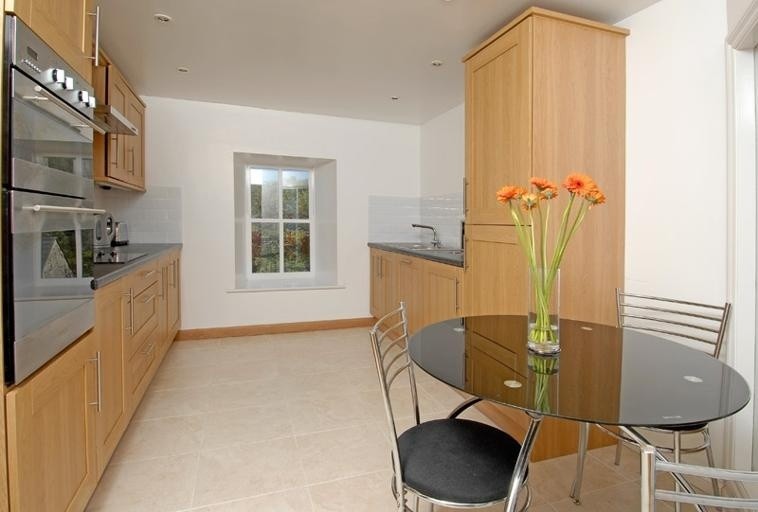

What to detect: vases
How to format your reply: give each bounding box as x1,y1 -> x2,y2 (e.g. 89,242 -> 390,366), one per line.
527,265 -> 561,355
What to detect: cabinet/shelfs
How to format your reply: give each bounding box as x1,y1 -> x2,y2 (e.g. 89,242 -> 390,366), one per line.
459,5 -> 629,465
93,40 -> 147,195
1,327 -> 102,511
1,0 -> 100,84
369,248 -> 462,353
94,250 -> 181,471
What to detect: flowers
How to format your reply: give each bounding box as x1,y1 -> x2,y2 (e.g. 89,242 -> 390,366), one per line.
496,175 -> 606,344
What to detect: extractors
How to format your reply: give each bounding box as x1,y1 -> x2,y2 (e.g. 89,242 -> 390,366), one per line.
94,104 -> 138,136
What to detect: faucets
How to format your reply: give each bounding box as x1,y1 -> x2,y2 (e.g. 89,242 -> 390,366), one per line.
411,222 -> 440,247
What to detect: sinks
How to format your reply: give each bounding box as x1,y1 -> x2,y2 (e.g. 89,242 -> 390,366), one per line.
383,243 -> 457,250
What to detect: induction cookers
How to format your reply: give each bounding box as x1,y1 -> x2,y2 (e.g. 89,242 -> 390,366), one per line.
95,251 -> 148,264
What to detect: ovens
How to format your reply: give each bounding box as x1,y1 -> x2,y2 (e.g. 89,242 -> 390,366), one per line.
2,12 -> 106,387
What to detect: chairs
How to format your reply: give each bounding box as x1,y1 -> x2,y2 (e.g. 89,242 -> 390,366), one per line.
640,446 -> 757,512
370,301 -> 534,510
569,286 -> 731,512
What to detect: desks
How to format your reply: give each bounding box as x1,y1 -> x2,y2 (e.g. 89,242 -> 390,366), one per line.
409,315 -> 754,512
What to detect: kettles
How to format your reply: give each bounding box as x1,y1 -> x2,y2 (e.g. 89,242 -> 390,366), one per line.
111,222 -> 128,247
